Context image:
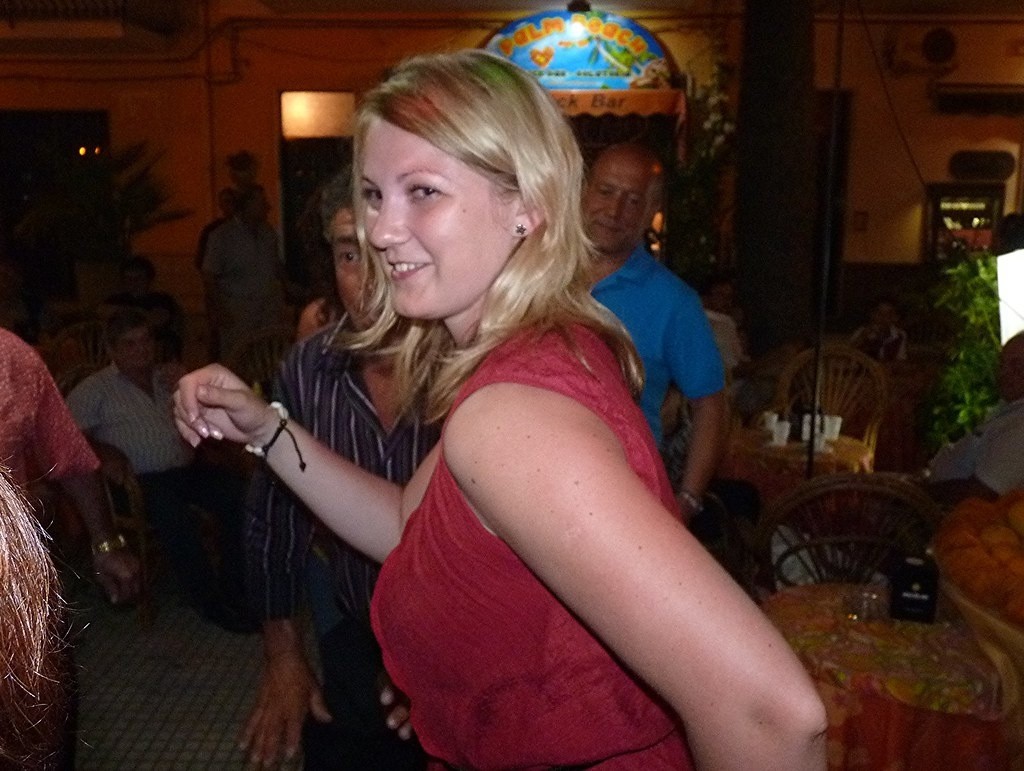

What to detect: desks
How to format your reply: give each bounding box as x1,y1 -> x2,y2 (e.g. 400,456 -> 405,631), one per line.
705,424 -> 870,566
836,357 -> 932,472
746,581 -> 1024,771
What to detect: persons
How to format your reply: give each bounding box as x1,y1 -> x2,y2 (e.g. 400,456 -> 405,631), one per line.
848,295 -> 906,365
579,141 -> 769,537
915,333 -> 1024,515
172,48 -> 828,771
0,154 -> 451,771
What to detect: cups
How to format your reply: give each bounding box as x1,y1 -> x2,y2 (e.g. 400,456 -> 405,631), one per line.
773,422 -> 791,446
822,415 -> 843,441
801,414 -> 821,441
844,586 -> 880,621
764,411 -> 778,429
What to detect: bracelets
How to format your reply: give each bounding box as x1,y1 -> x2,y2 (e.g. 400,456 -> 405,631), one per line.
244,401 -> 306,474
678,490 -> 703,514
91,535 -> 125,554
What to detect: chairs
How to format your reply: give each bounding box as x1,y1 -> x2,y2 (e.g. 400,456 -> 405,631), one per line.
757,473 -> 947,600
44,321 -> 110,401
81,439 -> 215,628
772,347 -> 887,460
226,323 -> 299,412
